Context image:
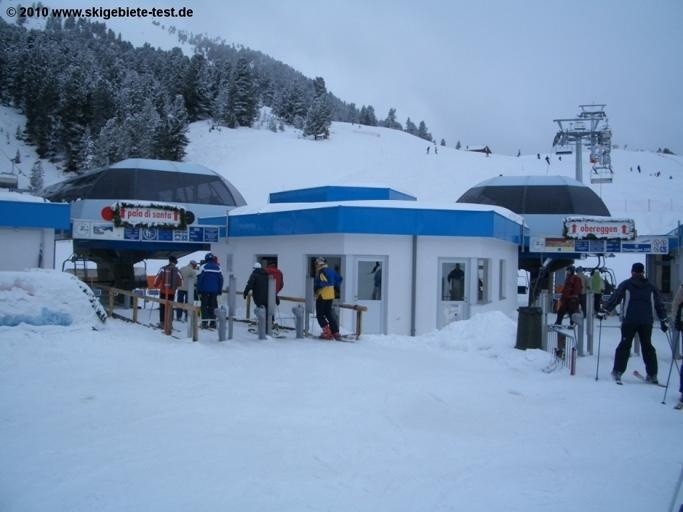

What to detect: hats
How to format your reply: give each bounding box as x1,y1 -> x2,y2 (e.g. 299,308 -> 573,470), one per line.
190,260 -> 196,266
254,263 -> 261,268
168,256 -> 176,264
314,257 -> 324,265
631,263 -> 643,273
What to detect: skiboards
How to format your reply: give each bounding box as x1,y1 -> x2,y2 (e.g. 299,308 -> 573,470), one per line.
248,321 -> 290,339
610,370 -> 683,410
149,320 -> 182,335
309,332 -> 360,345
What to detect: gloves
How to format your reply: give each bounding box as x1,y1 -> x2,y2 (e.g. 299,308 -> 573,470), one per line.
596,309 -> 605,320
660,318 -> 667,332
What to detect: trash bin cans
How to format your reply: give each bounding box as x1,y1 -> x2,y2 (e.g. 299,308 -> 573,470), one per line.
514,305 -> 543,350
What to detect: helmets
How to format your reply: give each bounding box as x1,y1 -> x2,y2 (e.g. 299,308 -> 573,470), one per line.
205,253 -> 214,262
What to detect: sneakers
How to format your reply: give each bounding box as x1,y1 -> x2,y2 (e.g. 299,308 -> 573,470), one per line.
319,325 -> 332,338
646,375 -> 656,381
611,370 -> 621,380
332,332 -> 340,340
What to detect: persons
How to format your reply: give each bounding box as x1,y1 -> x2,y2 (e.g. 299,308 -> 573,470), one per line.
195,252 -> 223,331
312,254 -> 344,340
552,265 -> 582,328
574,265 -> 593,318
262,259 -> 283,292
588,268 -> 607,315
595,261 -> 670,383
668,279 -> 682,402
176,259 -> 198,324
371,265 -> 382,300
443,262 -> 466,283
152,254 -> 182,332
241,261 -> 280,334
370,262 -> 380,273
